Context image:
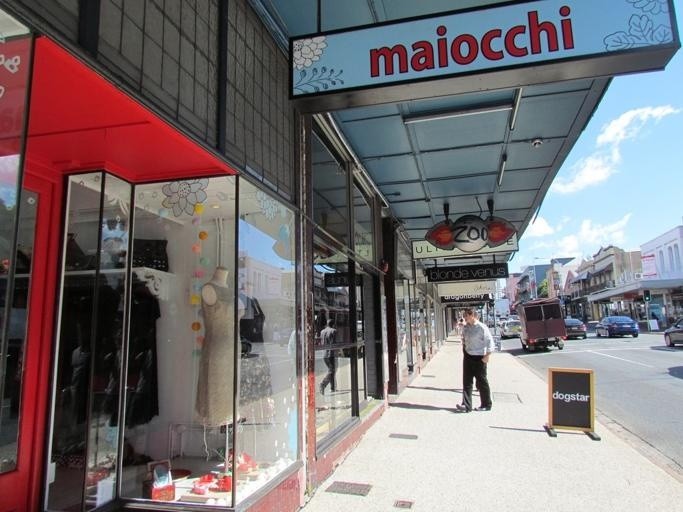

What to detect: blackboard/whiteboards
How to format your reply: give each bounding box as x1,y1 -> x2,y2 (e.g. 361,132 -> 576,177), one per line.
549,368 -> 594,432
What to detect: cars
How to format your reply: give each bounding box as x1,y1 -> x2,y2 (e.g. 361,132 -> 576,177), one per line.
357,320 -> 362,333
662,316 -> 682,348
272,326 -> 292,348
649,303 -> 663,320
489,308 -> 644,340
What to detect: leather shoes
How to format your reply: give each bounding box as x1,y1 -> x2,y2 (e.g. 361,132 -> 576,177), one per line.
319,383 -> 325,395
456,403 -> 469,413
475,406 -> 491,411
330,389 -> 335,392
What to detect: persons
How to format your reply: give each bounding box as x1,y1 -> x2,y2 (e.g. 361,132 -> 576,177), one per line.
195,267 -> 274,426
287,330 -> 296,380
458,319 -> 465,335
457,307 -> 494,413
318,319 -> 339,394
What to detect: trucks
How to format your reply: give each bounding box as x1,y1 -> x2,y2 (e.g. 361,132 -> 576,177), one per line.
515,296 -> 568,352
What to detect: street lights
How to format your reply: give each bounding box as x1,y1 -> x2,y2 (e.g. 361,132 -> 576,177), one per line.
532,256 -> 564,303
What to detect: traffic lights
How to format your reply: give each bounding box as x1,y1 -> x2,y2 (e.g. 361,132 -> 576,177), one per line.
642,289 -> 651,302
